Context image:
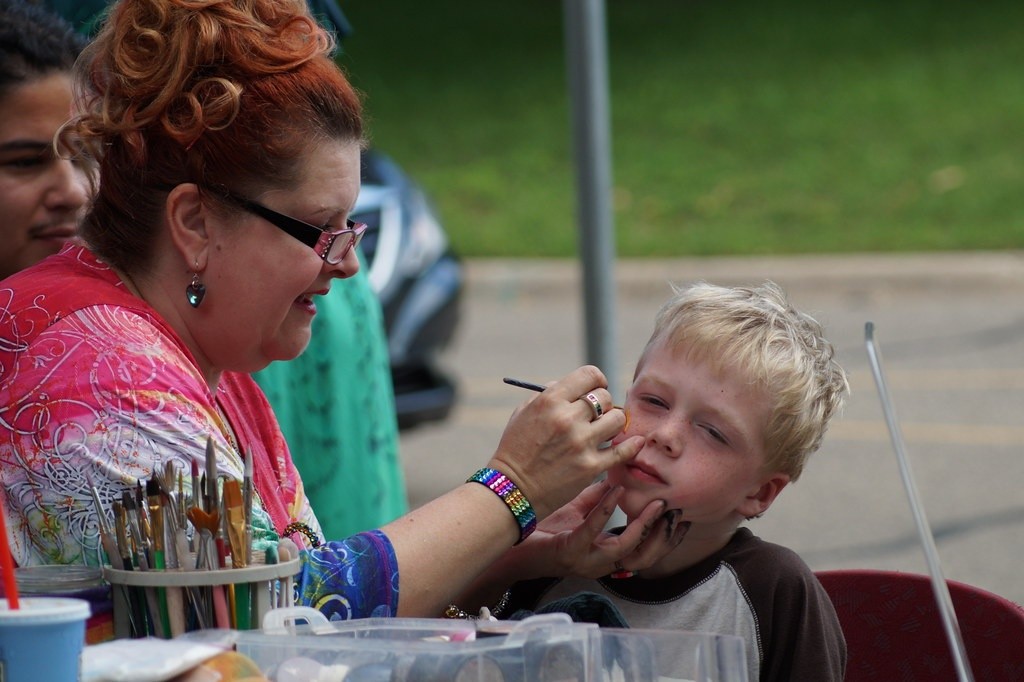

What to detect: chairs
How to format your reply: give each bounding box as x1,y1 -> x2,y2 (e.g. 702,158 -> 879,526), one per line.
807,569 -> 1023,681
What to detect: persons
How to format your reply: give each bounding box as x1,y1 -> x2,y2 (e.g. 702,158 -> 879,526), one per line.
1,0 -> 108,280
504,276 -> 848,682
0,0 -> 651,635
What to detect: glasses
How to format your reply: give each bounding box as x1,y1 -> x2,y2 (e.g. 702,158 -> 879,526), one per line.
187,177 -> 369,264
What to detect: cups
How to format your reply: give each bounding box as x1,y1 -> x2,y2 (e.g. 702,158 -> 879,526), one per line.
0,598 -> 91,682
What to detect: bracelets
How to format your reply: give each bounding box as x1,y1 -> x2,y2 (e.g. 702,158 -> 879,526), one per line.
444,590 -> 511,621
466,467 -> 538,546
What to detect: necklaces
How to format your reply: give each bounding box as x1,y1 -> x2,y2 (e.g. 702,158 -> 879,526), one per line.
216,409 -> 322,550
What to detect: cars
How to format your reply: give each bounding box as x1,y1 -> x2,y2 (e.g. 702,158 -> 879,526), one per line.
349,140 -> 463,432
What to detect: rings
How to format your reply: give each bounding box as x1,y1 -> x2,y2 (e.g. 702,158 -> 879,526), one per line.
578,392 -> 604,420
610,560 -> 639,581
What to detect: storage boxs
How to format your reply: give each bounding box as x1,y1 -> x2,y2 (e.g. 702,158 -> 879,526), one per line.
232,618 -> 751,681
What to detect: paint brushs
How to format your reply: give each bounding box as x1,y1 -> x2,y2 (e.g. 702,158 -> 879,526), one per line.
84,434 -> 255,644
502,377 -> 625,411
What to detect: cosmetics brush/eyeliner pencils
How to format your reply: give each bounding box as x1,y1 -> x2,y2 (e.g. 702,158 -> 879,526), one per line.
86,471 -> 122,569
112,433 -> 295,638
503,377 -> 624,412
